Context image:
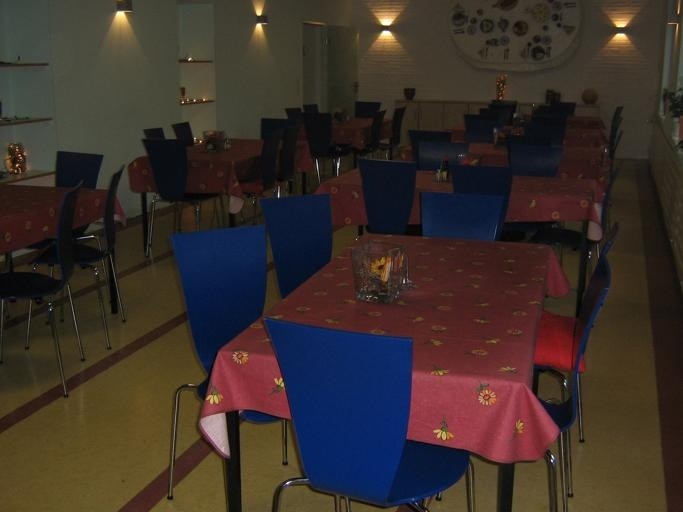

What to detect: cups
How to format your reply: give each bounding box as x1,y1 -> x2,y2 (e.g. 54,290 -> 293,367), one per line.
349,237 -> 408,305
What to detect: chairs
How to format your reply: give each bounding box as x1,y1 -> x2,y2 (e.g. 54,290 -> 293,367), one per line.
1,152 -> 126,350
128,96 -> 627,273
0,178 -> 85,397
168,192 -> 620,509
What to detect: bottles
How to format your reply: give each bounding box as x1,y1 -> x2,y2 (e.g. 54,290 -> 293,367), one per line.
439,154 -> 449,183
435,168 -> 442,184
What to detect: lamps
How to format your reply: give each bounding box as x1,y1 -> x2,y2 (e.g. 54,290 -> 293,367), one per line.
377,23 -> 393,34
114,0 -> 135,14
254,14 -> 268,25
614,25 -> 626,35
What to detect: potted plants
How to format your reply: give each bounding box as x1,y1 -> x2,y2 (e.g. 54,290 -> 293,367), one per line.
663,88 -> 682,123
